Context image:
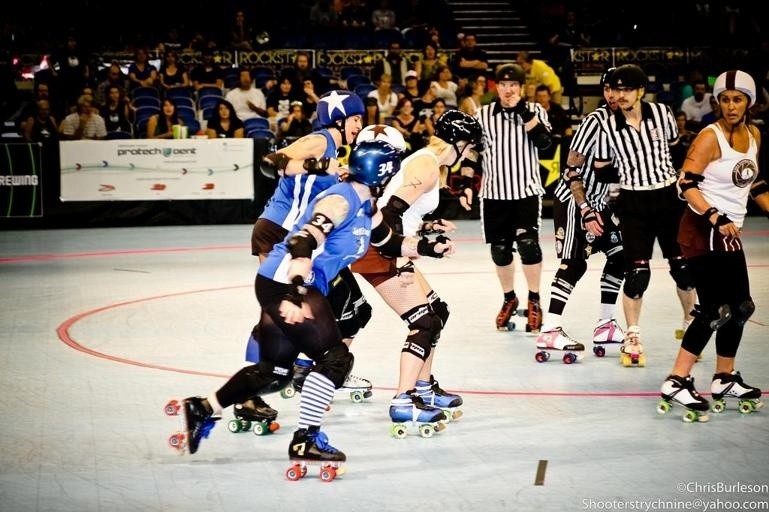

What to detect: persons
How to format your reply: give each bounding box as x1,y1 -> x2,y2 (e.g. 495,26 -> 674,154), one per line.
1,0 -> 769,217
588,59 -> 702,356
236,91 -> 367,418
343,109 -> 484,424
466,61 -> 550,332
329,121 -> 411,395
662,69 -> 769,408
182,139 -> 456,465
529,66 -> 626,353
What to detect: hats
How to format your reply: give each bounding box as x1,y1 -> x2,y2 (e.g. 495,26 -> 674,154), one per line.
405,70 -> 417,78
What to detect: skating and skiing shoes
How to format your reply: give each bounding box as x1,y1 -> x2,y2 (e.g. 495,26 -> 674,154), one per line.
536,319 -> 646,367
287,426 -> 346,482
165,395 -> 279,454
389,375 -> 463,439
496,295 -> 541,332
281,362 -> 372,403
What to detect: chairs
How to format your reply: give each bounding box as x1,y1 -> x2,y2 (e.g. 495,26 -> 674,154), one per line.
105,66 -> 407,139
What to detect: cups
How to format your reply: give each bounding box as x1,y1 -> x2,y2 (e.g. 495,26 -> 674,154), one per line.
171,123 -> 188,139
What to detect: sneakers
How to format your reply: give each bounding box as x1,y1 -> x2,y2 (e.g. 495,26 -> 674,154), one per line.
656,372 -> 764,422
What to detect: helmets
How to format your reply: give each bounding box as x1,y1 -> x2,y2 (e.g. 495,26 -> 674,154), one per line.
316,90 -> 483,187
494,63 -> 526,83
712,70 -> 756,109
600,64 -> 649,88
